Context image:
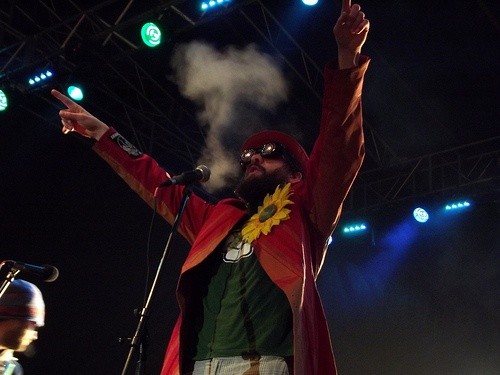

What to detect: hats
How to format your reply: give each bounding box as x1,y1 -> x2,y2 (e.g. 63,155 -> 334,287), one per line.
0,279 -> 46,327
242,129 -> 310,169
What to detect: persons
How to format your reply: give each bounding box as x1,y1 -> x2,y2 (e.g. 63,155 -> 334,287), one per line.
50,0 -> 372,375
0,280 -> 46,375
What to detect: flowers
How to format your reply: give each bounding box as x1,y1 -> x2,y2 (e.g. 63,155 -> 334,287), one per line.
240,182 -> 295,242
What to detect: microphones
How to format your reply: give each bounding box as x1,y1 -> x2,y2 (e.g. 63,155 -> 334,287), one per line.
158,165 -> 211,188
5,260 -> 59,281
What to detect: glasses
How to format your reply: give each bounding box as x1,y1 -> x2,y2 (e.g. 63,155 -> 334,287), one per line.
239,141 -> 296,173
